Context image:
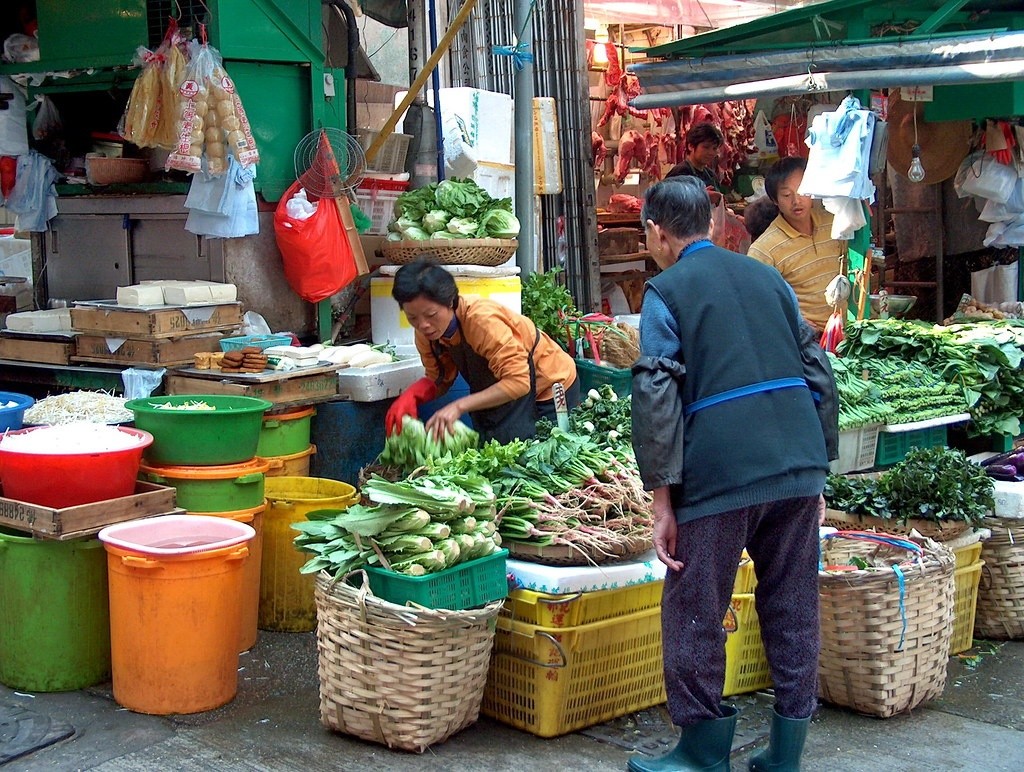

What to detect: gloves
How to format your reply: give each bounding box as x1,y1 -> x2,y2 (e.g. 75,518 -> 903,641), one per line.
385,376 -> 439,439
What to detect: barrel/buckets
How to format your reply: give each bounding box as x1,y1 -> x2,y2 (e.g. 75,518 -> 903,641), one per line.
0,395 -> 363,716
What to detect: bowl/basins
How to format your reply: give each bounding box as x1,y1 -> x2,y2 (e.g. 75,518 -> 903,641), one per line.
124,393 -> 274,465
0,424 -> 155,510
0,391 -> 35,434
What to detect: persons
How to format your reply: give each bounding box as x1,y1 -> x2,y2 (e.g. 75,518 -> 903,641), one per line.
387,263 -> 580,451
746,156 -> 849,343
627,174 -> 841,772
870,172 -> 920,318
744,194 -> 780,237
663,121 -> 724,206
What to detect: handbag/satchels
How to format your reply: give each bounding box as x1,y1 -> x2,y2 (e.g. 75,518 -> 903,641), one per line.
707,192 -> 727,248
273,134 -> 357,305
22,94 -> 59,141
746,109 -> 778,161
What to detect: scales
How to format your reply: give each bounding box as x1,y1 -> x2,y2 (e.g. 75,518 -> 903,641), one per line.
735,156 -> 767,196
869,293 -> 919,321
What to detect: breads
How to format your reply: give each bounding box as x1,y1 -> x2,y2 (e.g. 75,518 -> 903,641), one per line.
122,49 -> 252,176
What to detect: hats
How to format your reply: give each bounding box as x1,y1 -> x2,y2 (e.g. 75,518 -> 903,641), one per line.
883,85 -> 974,184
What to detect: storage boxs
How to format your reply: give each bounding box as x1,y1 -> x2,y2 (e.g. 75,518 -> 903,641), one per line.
829,424 -> 948,475
348,549 -> 775,738
0,77 -> 562,407
948,541 -> 985,656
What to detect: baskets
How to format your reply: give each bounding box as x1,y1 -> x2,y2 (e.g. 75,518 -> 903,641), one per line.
812,528 -> 955,719
355,550 -> 509,611
822,468 -> 969,541
313,568 -> 504,754
85,154 -> 146,184
380,239 -> 519,267
357,460 -> 655,565
974,515 -> 1024,641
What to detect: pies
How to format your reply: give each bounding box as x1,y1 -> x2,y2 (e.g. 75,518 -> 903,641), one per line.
218,347 -> 268,373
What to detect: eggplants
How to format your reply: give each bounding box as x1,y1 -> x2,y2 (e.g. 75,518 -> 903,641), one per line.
981,444 -> 1024,482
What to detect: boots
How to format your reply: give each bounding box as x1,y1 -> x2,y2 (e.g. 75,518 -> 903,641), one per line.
749,702 -> 812,772
627,705 -> 739,772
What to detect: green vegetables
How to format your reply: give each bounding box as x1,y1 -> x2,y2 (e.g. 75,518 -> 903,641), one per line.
821,318 -> 1024,437
823,445 -> 996,532
517,266 -> 640,367
287,385 -> 655,587
384,177 -> 521,241
